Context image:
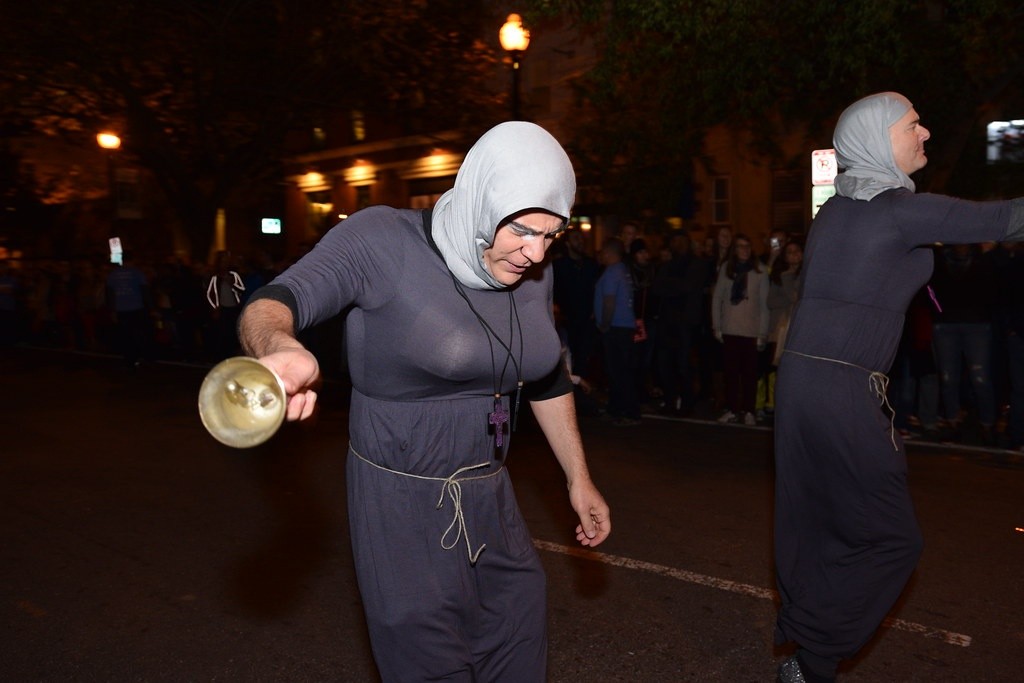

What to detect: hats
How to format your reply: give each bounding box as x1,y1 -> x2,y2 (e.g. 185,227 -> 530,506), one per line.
630,238 -> 646,256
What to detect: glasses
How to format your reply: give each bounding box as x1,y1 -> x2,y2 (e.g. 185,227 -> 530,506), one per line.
734,243 -> 751,249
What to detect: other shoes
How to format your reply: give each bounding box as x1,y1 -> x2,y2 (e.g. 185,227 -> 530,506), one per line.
642,398 -> 665,412
753,409 -> 764,422
576,394 -> 606,417
595,412 -> 616,422
744,411 -> 756,426
892,408 -> 1024,456
777,656 -> 806,683
666,407 -> 684,418
612,416 -> 640,427
763,407 -> 774,418
717,410 -> 740,425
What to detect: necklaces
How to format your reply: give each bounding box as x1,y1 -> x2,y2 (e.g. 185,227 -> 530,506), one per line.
450,273 -> 524,448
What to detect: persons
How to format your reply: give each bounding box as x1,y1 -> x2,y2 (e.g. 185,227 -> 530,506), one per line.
242,121 -> 611,683
0,250 -> 275,363
553,220 -> 1024,463
594,236 -> 642,426
774,92 -> 1024,683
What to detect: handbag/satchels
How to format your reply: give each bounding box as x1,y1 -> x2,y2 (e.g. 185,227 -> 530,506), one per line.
629,318 -> 647,343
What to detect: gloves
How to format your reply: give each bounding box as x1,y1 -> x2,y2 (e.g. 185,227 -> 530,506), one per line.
713,328 -> 724,343
756,337 -> 767,352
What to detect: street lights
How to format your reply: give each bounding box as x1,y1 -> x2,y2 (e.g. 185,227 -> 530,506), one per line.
499,12 -> 530,121
